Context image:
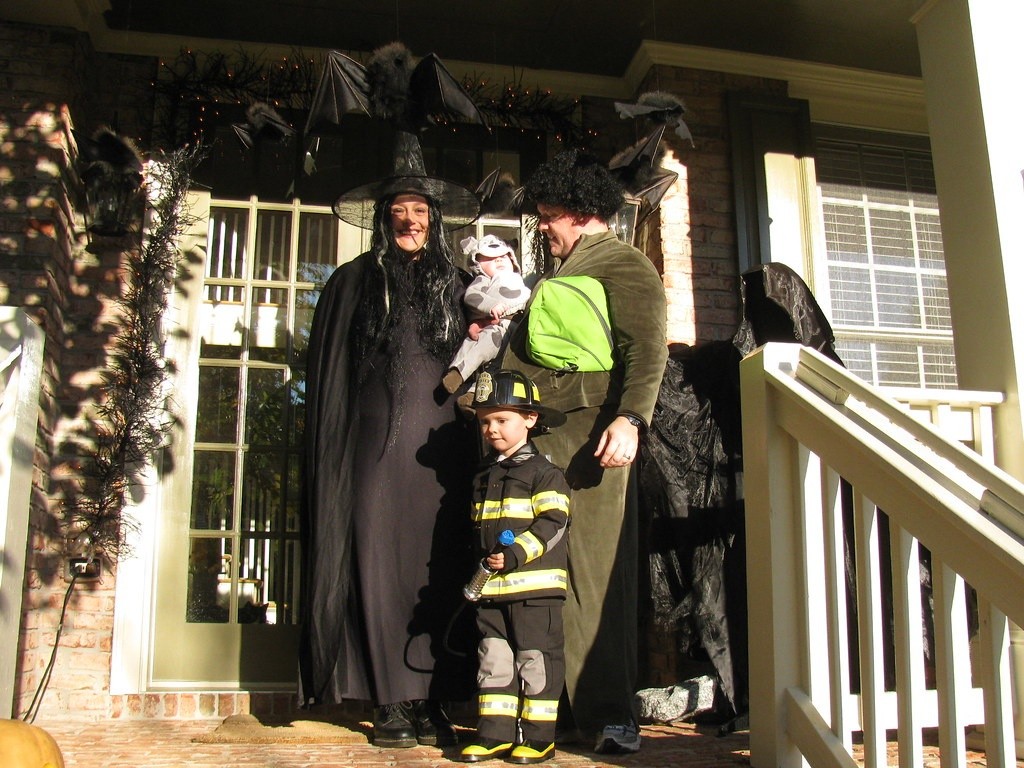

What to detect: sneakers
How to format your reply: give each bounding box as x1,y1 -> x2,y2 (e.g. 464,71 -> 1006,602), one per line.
595,719 -> 641,753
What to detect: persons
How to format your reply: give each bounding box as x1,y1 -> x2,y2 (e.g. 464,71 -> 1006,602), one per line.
443,234 -> 531,407
297,190 -> 475,748
469,152 -> 671,754
459,369 -> 572,763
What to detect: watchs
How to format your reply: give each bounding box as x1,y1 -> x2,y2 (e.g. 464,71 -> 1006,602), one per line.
626,415 -> 642,430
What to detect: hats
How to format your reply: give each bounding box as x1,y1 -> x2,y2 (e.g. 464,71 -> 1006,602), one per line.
472,369 -> 567,428
336,130 -> 485,232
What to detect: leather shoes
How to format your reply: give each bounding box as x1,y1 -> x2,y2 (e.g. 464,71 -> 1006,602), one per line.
460,739 -> 514,762
511,740 -> 558,763
374,701 -> 459,748
